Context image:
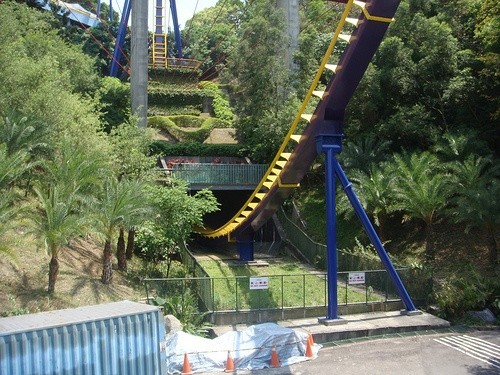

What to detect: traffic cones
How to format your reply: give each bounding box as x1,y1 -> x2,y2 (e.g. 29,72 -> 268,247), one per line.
224,349 -> 236,373
304,337 -> 314,358
269,344 -> 281,368
180,353 -> 194,375
310,334 -> 314,347
